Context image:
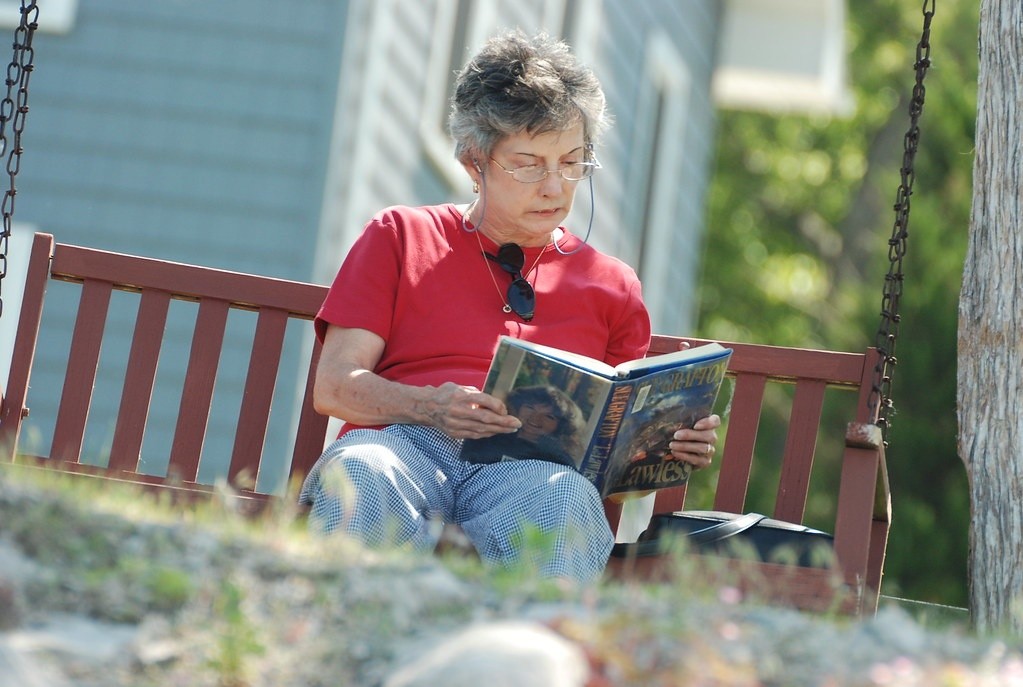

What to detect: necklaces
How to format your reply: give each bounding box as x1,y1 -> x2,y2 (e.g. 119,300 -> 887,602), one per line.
470,216 -> 551,313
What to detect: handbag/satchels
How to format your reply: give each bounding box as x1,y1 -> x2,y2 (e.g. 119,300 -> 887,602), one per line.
614,509 -> 834,567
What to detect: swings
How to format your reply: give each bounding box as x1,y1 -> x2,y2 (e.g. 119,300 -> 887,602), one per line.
1,0 -> 943,616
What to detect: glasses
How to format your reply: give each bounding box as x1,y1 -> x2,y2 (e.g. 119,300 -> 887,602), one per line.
483,242 -> 535,323
489,144 -> 600,184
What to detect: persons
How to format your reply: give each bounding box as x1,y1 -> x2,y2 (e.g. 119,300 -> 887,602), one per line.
300,33 -> 721,584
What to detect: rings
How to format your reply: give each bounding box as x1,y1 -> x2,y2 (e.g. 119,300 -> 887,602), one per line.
707,443 -> 710,455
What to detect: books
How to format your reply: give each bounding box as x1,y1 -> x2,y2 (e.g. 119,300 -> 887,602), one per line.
460,335 -> 734,499
